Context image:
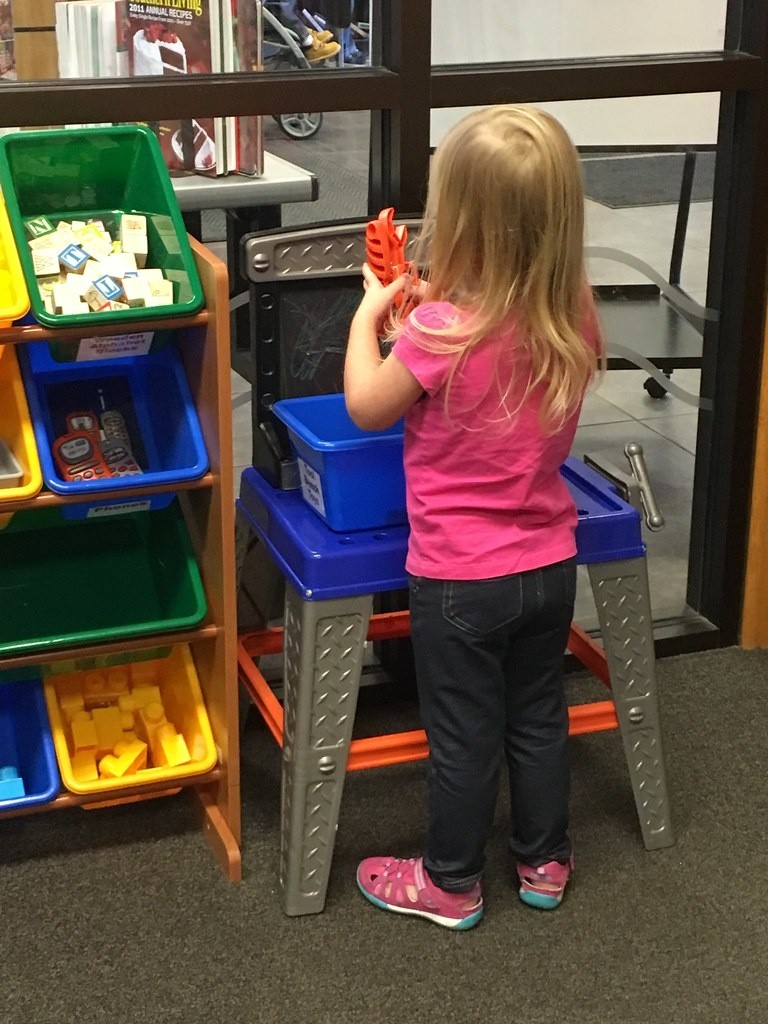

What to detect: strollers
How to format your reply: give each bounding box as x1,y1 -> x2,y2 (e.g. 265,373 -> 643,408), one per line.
259,0 -> 325,139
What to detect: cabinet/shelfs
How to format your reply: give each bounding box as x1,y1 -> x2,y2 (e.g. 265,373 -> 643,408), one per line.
0,232 -> 250,883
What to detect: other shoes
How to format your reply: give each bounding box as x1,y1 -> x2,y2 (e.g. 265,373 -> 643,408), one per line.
302,27 -> 340,63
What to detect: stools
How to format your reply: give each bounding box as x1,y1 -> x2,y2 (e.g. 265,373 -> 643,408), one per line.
236,454 -> 677,916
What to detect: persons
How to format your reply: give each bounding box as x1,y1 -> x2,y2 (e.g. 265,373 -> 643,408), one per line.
341,101 -> 614,934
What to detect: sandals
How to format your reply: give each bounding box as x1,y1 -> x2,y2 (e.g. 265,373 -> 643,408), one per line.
356,854 -> 484,928
517,851 -> 573,910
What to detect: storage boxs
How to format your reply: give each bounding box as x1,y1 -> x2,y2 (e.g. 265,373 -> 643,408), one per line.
1,660 -> 65,815
266,383 -> 423,538
43,638 -> 224,794
3,121 -> 206,327
0,516 -> 216,666
0,341 -> 44,503
16,341 -> 217,499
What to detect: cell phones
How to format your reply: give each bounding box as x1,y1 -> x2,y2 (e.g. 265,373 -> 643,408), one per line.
52,389 -> 144,482
